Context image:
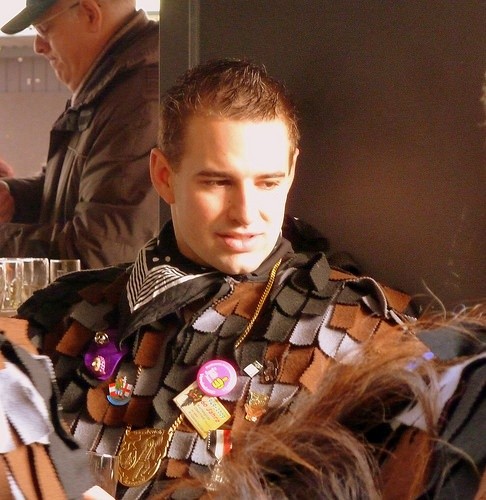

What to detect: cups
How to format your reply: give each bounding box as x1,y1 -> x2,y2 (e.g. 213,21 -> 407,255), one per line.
1,257 -> 24,315
47,260 -> 81,283
20,256 -> 49,298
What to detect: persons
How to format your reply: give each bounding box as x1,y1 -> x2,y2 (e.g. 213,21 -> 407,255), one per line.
0,57 -> 425,500
0,0 -> 160,268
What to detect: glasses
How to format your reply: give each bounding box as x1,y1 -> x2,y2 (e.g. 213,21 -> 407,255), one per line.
30,1 -> 93,36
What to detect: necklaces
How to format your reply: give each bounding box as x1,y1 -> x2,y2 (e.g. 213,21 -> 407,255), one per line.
116,258 -> 284,488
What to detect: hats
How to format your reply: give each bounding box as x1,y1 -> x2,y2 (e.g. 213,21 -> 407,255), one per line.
0,1 -> 57,34
